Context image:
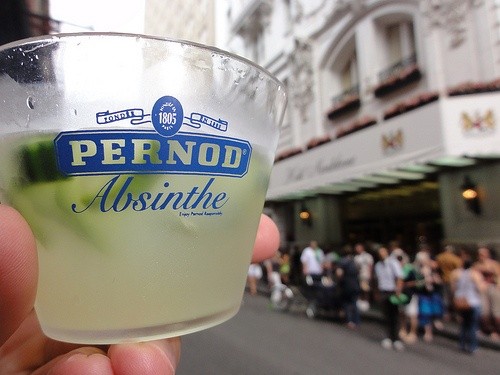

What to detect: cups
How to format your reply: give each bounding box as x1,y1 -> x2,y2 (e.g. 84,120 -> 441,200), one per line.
0,32 -> 288,345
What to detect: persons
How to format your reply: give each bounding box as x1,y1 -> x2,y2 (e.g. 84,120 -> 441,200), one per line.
246,236 -> 500,354
0,202 -> 281,375
